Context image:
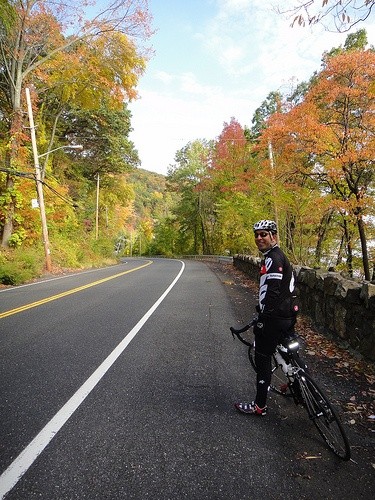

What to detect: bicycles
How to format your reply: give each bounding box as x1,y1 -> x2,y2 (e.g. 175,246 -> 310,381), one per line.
230,316 -> 350,462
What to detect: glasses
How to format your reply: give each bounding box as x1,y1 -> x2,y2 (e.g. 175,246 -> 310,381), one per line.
254,232 -> 270,238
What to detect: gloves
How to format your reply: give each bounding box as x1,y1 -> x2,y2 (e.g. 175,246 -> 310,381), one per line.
255,304 -> 261,314
253,317 -> 267,339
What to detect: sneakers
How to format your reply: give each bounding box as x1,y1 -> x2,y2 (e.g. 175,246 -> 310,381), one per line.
278,383 -> 293,394
235,401 -> 268,416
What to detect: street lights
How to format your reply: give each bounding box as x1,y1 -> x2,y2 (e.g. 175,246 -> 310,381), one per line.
25,87 -> 83,273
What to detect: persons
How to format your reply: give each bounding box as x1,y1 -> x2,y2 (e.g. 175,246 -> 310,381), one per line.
234,219 -> 330,419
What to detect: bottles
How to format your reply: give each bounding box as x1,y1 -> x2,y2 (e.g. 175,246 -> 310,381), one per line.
273,349 -> 293,376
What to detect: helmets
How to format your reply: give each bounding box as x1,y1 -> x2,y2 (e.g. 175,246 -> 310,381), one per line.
253,219 -> 277,231
